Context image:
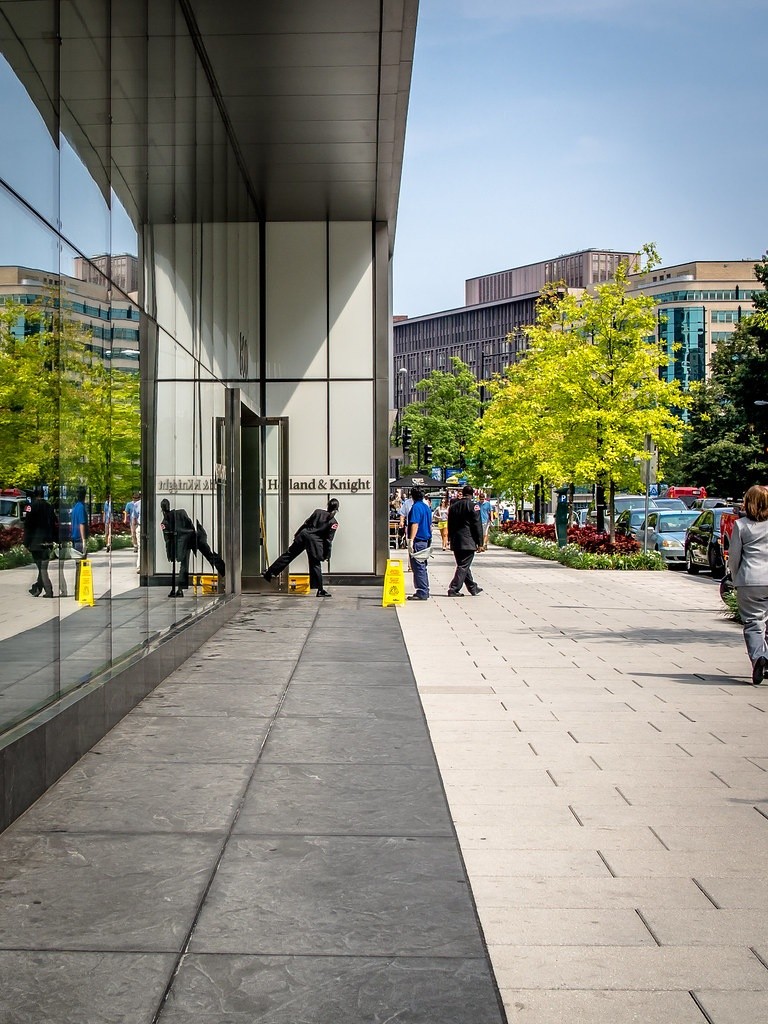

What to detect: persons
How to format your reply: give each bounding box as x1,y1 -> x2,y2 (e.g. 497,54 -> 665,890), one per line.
406,486 -> 431,601
447,486 -> 484,597
728,485 -> 768,685
72,486 -> 87,599
105,495 -> 112,552
264,498 -> 340,596
51,492 -> 70,596
24,488 -> 55,597
160,497 -> 225,597
124,493 -> 141,574
390,490 -> 503,553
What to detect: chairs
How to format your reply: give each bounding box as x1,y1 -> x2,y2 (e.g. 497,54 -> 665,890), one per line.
660,522 -> 669,532
389,523 -> 399,551
631,515 -> 640,525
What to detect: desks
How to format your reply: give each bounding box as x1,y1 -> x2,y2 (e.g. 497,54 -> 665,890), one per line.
389,520 -> 401,523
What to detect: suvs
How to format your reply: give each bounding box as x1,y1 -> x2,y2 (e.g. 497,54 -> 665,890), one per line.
0,496 -> 123,531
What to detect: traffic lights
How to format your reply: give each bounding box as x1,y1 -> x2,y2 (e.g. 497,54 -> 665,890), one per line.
402,426 -> 412,453
424,444 -> 432,464
460,443 -> 466,468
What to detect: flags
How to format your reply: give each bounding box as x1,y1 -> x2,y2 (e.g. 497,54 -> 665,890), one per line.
721,511 -> 739,559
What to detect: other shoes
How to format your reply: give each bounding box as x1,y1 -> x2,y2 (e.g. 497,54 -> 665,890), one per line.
133,546 -> 138,553
763,671 -> 768,680
407,593 -> 430,600
29,589 -> 39,597
448,592 -> 464,597
442,546 -> 445,551
473,587 -> 483,596
171,591 -> 183,597
263,571 -> 272,583
481,547 -> 485,552
43,593 -> 54,598
317,590 -> 331,597
753,655 -> 765,685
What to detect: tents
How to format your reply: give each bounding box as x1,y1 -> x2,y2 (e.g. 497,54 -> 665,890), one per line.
389,473 -> 447,487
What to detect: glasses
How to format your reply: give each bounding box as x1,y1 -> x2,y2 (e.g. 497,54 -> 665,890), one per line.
479,499 -> 485,500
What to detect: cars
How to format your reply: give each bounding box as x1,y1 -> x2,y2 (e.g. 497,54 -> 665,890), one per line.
545,486 -> 743,577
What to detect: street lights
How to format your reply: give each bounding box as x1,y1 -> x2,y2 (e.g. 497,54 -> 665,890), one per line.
479,348 -> 544,469
396,368 -> 408,480
